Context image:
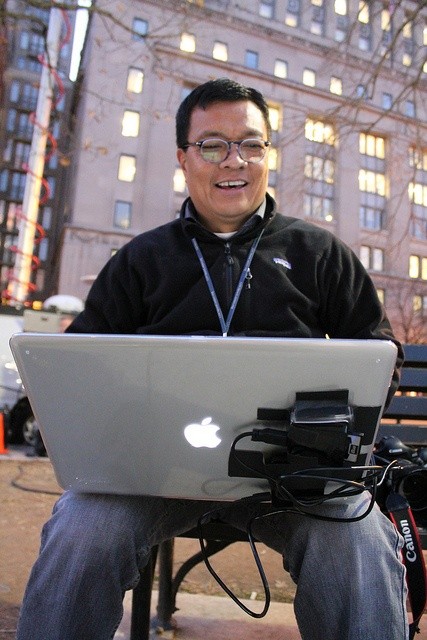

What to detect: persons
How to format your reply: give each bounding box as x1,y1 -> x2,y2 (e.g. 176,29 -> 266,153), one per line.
14,79 -> 411,640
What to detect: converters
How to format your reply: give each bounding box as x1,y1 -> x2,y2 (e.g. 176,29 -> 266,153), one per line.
272,404 -> 364,508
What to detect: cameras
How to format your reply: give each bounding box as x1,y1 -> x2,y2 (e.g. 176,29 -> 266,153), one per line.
367,435 -> 427,512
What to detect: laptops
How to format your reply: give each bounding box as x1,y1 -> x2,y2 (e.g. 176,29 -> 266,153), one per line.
8,332 -> 398,501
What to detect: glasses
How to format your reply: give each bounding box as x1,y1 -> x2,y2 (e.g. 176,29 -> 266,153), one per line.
184,139 -> 271,164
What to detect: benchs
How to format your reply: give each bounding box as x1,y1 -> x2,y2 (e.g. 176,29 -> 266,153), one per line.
33,342 -> 427,640
